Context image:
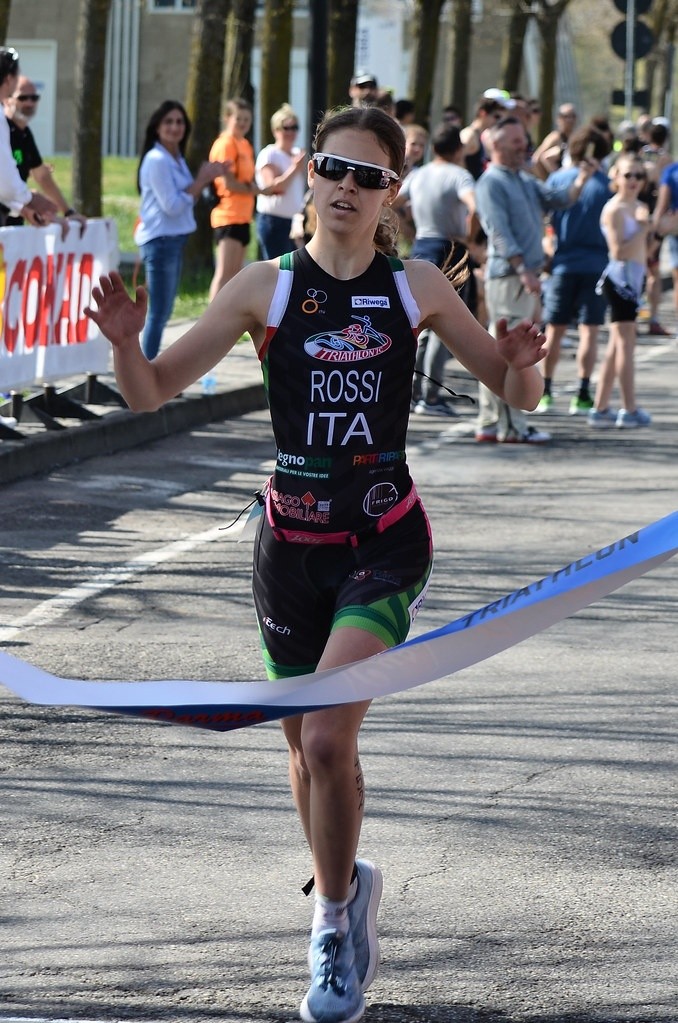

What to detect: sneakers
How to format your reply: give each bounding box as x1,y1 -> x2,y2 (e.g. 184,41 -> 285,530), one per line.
569,396 -> 595,415
587,407 -> 619,429
299,924 -> 366,1023
351,858 -> 383,991
520,395 -> 553,415
616,406 -> 651,429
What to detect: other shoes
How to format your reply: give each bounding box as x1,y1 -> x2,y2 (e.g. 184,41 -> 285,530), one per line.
408,397 -> 463,418
475,422 -> 553,441
650,321 -> 671,336
0,415 -> 17,430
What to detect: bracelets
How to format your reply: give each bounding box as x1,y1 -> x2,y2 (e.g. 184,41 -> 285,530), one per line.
244,182 -> 251,194
515,263 -> 529,276
574,180 -> 584,192
64,208 -> 77,217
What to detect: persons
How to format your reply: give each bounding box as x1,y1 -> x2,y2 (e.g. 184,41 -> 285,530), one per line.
254,103 -> 308,261
84,109 -> 549,1023
348,74 -> 678,444
208,97 -> 255,303
133,101 -> 233,397
0,47 -> 58,429
0,76 -> 87,237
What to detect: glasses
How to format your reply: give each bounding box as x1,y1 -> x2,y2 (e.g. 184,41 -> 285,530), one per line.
17,93 -> 39,102
283,125 -> 299,131
623,172 -> 645,180
311,153 -> 401,190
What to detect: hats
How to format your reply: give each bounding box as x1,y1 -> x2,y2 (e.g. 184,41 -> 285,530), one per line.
350,71 -> 378,87
